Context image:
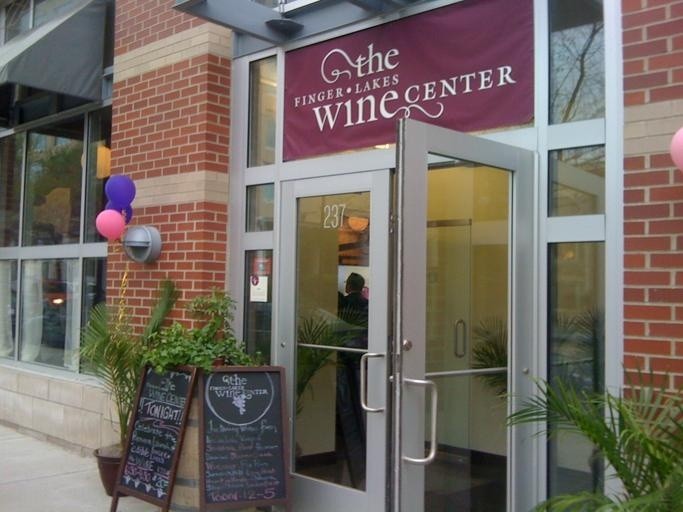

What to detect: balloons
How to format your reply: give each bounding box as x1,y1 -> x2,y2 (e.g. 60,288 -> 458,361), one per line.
670,129 -> 683,171
105,201 -> 133,224
96,209 -> 124,240
105,175 -> 136,207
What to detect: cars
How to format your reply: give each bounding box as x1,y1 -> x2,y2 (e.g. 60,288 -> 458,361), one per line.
10,279 -> 104,347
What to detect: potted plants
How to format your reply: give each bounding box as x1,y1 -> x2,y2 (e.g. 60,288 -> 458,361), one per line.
68,276 -> 185,498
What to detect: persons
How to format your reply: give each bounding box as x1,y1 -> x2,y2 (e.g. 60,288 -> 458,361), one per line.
338,271 -> 370,441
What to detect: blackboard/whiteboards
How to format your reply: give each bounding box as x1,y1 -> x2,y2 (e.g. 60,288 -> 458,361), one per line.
108,361 -> 199,508
195,365 -> 293,512
